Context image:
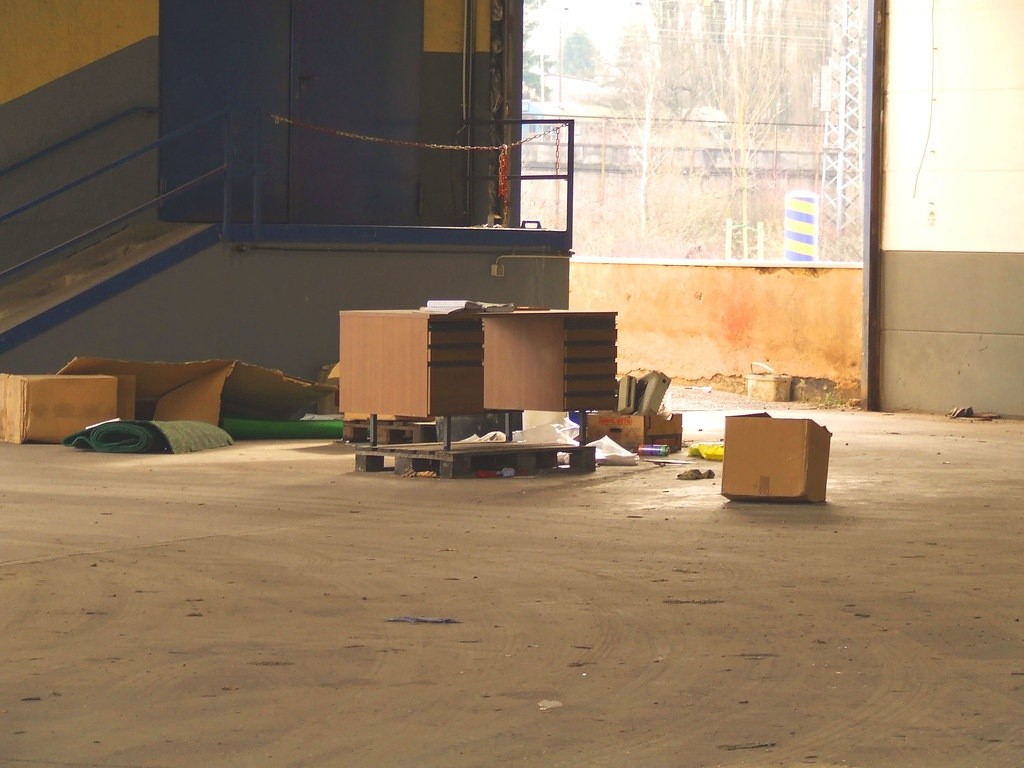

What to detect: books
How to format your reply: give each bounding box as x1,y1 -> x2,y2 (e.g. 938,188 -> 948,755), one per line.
475,302 -> 514,312
413,300 -> 482,315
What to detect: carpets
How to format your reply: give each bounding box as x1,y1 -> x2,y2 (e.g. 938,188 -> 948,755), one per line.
220,416 -> 344,439
64,421 -> 232,455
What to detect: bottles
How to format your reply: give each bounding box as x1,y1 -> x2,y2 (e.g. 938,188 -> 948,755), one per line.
637,444 -> 670,456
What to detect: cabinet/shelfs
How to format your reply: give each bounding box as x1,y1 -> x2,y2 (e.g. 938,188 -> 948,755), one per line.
339,309 -> 524,450
478,311 -> 620,442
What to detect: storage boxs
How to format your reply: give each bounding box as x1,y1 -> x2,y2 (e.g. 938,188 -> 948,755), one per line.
522,410 -> 569,431
587,412 -> 683,453
618,371 -> 671,415
722,413 -> 832,504
0,372 -> 136,444
317,364 -> 343,416
